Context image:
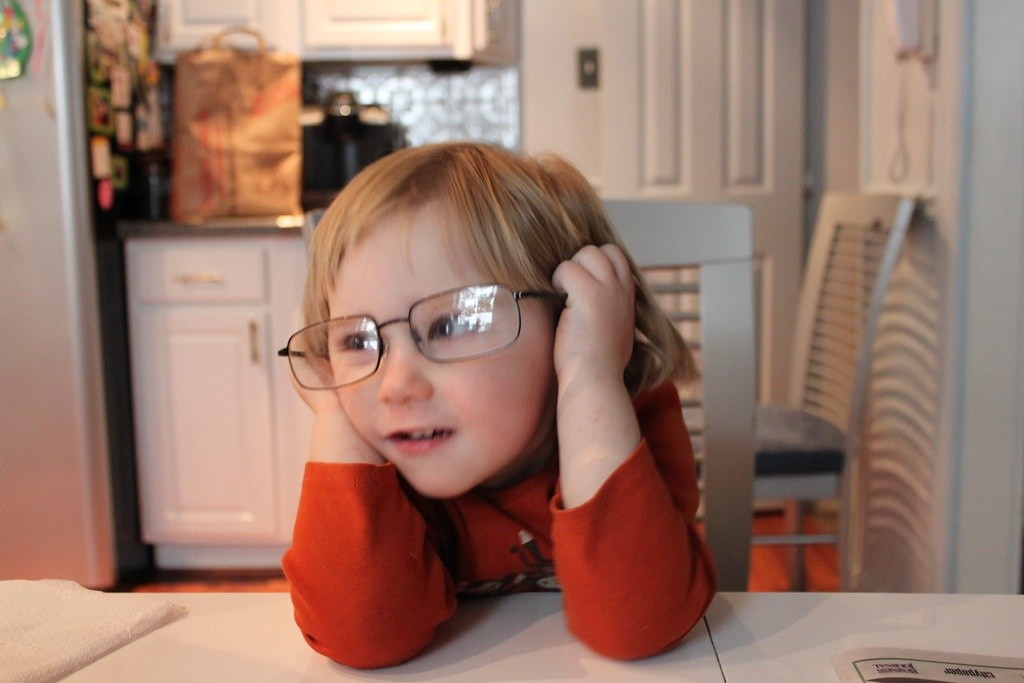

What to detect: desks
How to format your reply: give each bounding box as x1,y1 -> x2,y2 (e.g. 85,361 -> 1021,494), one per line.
0,578 -> 1024,683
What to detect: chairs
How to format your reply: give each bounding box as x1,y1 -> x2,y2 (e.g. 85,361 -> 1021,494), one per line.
602,196 -> 756,593
686,192 -> 915,592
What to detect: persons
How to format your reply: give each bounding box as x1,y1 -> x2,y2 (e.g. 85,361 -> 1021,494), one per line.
283,139 -> 716,672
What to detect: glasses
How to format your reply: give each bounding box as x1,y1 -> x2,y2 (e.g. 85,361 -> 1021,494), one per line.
277,281 -> 554,389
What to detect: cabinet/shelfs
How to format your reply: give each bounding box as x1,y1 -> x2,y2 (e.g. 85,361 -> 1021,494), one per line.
128,239 -> 310,574
517,0 -> 808,194
151,0 -> 520,68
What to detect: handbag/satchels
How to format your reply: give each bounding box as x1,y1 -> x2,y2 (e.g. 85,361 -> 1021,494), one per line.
306,91 -> 392,189
172,26 -> 305,225
